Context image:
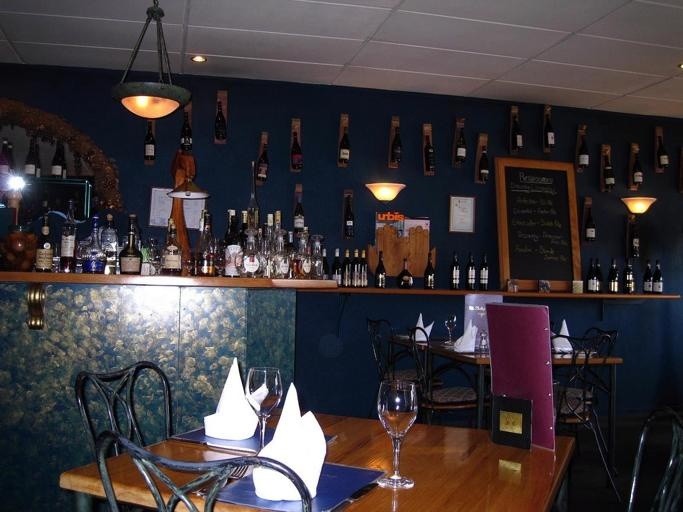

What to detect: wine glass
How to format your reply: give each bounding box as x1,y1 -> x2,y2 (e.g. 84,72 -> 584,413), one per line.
441,312 -> 459,346
376,378 -> 420,491
243,366 -> 283,453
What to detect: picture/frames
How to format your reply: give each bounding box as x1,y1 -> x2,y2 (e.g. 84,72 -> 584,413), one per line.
494,155 -> 582,292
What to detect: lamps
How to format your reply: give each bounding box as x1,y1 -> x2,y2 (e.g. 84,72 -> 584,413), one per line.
110,1 -> 190,121
621,197 -> 657,216
167,176 -> 210,200
365,181 -> 406,205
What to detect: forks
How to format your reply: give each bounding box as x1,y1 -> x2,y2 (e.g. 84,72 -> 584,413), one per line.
194,462 -> 250,499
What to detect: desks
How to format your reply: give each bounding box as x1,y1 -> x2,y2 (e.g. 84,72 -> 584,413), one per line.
382,333 -> 624,464
59,411 -> 576,512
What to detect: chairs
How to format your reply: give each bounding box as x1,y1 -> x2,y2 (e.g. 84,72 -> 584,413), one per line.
550,328 -> 628,504
627,407 -> 683,512
75,360 -> 174,460
94,430 -> 313,512
368,319 -> 476,424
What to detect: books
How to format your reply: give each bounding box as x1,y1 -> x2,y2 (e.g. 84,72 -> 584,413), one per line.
462,292 -> 503,347
485,302 -> 556,451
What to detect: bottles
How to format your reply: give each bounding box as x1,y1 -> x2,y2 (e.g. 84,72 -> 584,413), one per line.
453,127 -> 469,164
246,160 -> 259,228
344,193 -> 355,238
631,220 -> 641,257
451,248 -> 460,290
603,154 -> 614,188
290,130 -> 304,170
477,330 -> 488,355
477,145 -> 488,182
144,120 -> 155,161
0,136 -> 66,179
585,254 -> 663,294
214,100 -> 227,141
292,192 -> 305,231
59,198 -> 80,274
256,142 -> 270,181
424,139 -> 435,172
422,252 -> 435,290
578,138 -> 590,169
374,249 -> 386,290
179,111 -> 193,152
656,135 -> 669,169
390,126 -> 402,165
337,126 -> 351,164
581,194 -> 597,242
396,256 -> 414,289
476,252 -> 489,290
632,152 -> 643,185
79,207 -> 367,289
466,251 -> 476,291
511,114 -> 524,149
544,116 -> 555,148
35,207 -> 55,272
